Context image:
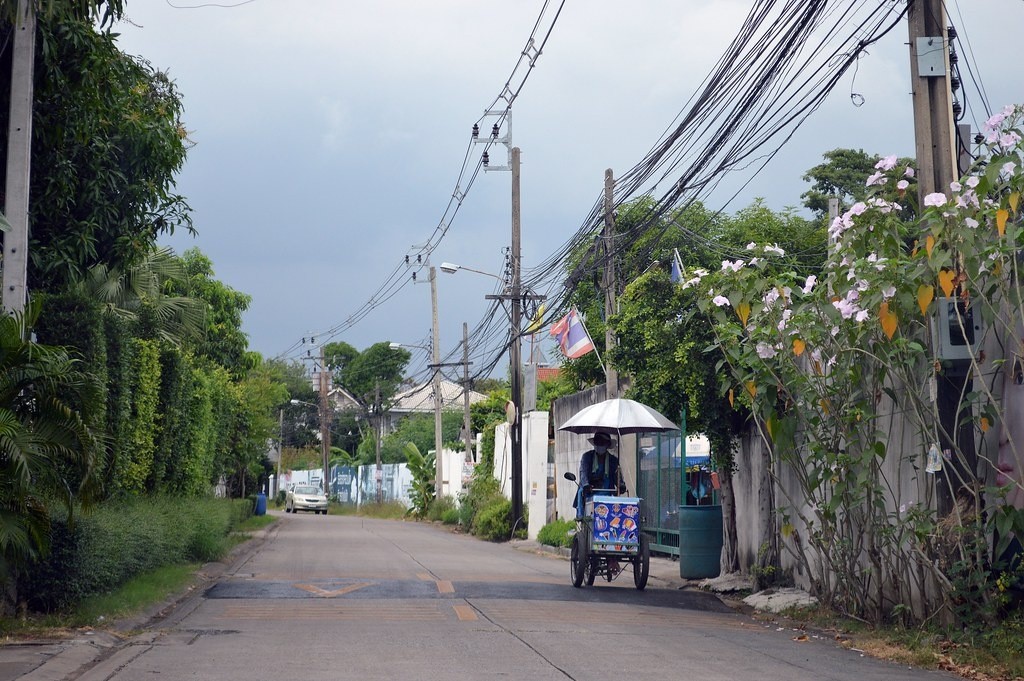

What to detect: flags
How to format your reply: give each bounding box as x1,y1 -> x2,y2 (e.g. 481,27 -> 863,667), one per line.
549,308 -> 594,360
524,304 -> 544,335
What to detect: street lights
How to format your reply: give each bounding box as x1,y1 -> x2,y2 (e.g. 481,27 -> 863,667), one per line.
388,342 -> 444,500
440,262 -> 522,521
289,398 -> 330,498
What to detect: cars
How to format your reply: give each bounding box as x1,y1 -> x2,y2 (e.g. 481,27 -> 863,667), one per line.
286,485 -> 328,515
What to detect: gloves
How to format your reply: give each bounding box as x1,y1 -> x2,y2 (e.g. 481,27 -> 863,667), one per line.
620,486 -> 625,493
583,486 -> 591,493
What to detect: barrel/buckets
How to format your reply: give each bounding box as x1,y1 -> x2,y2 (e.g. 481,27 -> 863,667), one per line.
255,493 -> 266,515
678,504 -> 724,579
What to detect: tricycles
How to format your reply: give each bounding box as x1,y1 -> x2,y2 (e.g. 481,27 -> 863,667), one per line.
564,472 -> 650,590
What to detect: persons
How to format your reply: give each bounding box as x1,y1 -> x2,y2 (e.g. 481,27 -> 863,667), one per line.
572,431 -> 626,577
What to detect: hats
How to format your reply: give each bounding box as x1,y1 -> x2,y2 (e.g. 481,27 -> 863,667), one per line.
588,429 -> 616,450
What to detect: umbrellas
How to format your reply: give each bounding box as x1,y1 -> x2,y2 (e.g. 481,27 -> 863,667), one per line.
556,389 -> 681,498
639,432 -> 712,505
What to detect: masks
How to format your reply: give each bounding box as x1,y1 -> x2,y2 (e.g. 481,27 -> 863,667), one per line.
594,446 -> 607,455
